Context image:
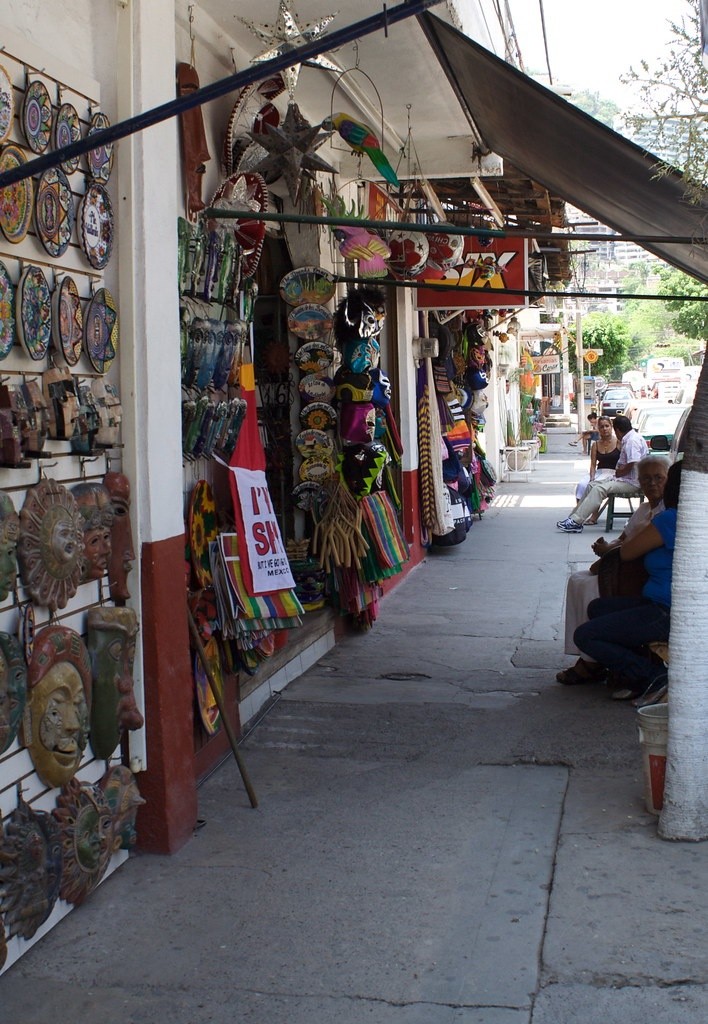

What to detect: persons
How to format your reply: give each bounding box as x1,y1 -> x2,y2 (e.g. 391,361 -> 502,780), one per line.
552,412 -> 682,707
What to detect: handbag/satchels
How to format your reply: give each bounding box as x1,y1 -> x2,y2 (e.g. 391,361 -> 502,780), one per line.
208,532 -> 306,677
435,324 -> 498,547
356,490 -> 412,584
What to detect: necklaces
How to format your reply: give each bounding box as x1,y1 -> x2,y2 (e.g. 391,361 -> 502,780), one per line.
600,439 -> 611,449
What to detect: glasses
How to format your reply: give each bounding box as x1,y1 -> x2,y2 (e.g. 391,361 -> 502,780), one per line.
640,475 -> 666,484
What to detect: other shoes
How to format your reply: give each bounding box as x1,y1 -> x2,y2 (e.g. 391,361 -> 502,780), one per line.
631,683 -> 668,707
556,668 -> 607,685
569,441 -> 578,446
612,689 -> 635,700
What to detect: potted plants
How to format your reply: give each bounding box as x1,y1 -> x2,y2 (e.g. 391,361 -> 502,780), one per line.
505,408 -> 538,471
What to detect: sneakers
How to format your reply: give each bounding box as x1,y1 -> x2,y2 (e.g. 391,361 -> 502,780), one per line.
558,518 -> 582,532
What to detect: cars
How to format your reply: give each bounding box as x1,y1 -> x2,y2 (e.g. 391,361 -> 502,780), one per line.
600,356 -> 702,460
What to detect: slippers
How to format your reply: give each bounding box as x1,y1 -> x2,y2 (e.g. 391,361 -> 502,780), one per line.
584,519 -> 597,525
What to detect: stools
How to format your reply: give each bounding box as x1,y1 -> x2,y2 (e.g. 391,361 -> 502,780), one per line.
606,492 -> 645,534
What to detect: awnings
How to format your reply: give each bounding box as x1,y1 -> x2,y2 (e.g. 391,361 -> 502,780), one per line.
419,4 -> 708,290
